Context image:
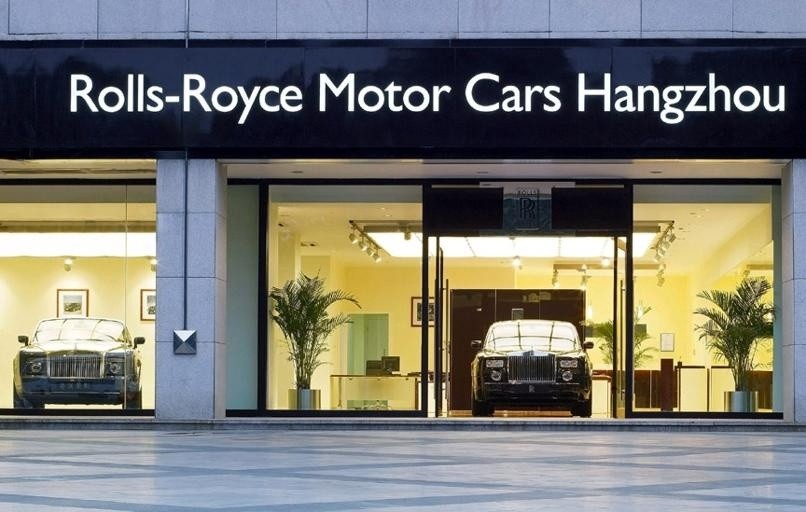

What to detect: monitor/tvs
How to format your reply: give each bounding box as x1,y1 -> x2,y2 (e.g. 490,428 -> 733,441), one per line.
381,355 -> 400,371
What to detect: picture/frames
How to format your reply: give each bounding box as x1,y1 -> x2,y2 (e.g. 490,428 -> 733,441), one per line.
56,288 -> 88,318
661,333 -> 675,352
140,289 -> 155,321
412,296 -> 435,327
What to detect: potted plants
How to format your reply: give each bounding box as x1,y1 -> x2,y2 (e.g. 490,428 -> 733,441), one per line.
265,266 -> 361,409
693,270 -> 779,413
579,300 -> 659,408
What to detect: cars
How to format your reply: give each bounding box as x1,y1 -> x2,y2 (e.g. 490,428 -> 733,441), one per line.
13,317 -> 146,410
469,318 -> 594,417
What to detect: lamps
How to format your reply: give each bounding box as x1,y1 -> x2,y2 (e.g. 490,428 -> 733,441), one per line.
350,220 -> 383,263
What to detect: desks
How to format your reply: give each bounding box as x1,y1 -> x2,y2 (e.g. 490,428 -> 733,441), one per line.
330,372 -> 419,411
